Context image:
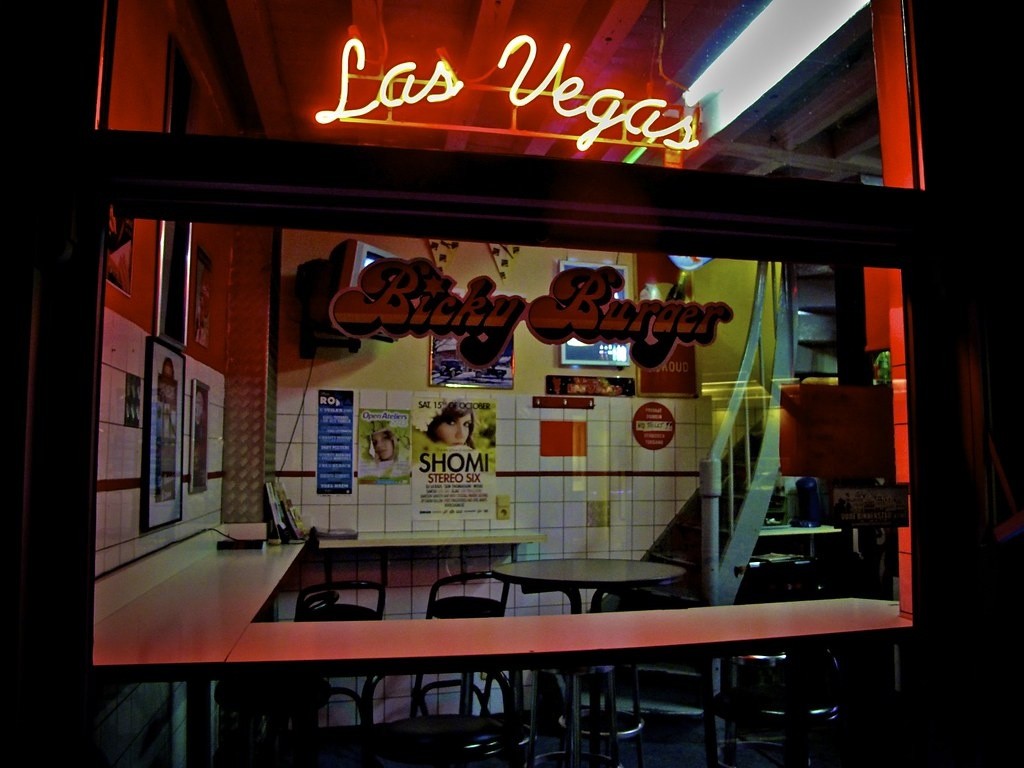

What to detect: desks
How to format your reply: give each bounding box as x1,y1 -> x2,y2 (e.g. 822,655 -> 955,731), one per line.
490,558 -> 688,767
92,534 -> 913,768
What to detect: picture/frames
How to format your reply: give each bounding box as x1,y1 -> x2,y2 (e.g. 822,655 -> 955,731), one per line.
106,205 -> 211,534
560,260 -> 631,367
429,333 -> 515,390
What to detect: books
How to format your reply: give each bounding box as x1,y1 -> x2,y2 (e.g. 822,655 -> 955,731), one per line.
266,480 -> 306,540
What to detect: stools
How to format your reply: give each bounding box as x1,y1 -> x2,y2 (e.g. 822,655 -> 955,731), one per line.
194,568 -> 847,768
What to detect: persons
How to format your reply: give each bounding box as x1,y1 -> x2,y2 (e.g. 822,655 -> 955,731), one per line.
371,429 -> 395,462
427,402 -> 475,449
